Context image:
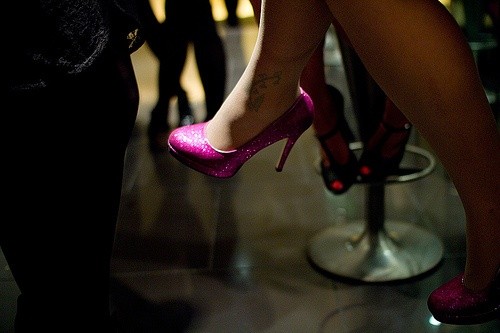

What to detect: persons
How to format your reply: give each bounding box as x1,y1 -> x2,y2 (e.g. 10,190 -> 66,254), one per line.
250,0 -> 414,196
155,0 -> 500,326
1,0 -> 203,333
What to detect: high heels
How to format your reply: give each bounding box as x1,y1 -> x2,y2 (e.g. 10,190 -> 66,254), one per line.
168,91 -> 317,176
358,117 -> 414,186
322,86 -> 359,194
427,273 -> 500,323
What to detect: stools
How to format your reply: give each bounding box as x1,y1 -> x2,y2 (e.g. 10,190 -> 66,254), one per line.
304,17 -> 447,284
